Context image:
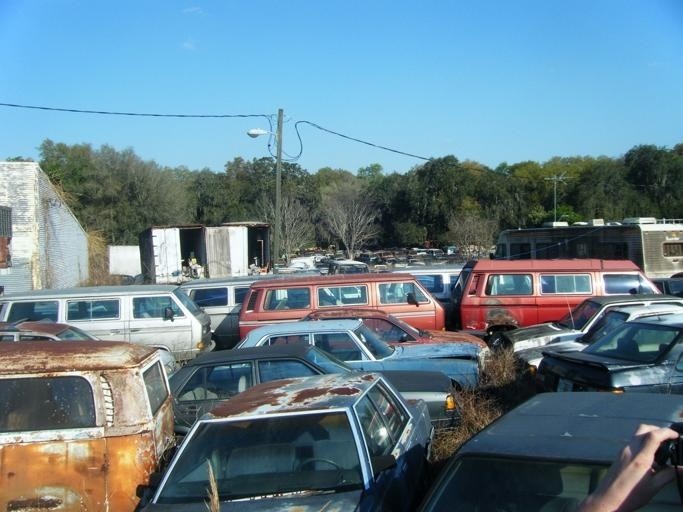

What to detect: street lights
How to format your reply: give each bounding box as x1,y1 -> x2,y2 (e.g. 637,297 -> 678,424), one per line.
248,127 -> 282,273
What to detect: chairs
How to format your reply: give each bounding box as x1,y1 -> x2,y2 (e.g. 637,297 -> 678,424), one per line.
512,461 -> 680,510
226,425 -> 362,475
614,338 -> 671,362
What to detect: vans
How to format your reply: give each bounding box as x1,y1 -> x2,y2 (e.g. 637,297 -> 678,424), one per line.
0,285 -> 216,361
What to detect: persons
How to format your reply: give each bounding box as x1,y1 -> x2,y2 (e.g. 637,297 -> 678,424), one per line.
575,421 -> 682,511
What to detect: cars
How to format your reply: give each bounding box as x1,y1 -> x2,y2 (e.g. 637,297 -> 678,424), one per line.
0,322 -> 181,392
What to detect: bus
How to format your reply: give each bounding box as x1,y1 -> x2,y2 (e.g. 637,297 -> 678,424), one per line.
490,216 -> 683,295
409,249 -> 444,256
